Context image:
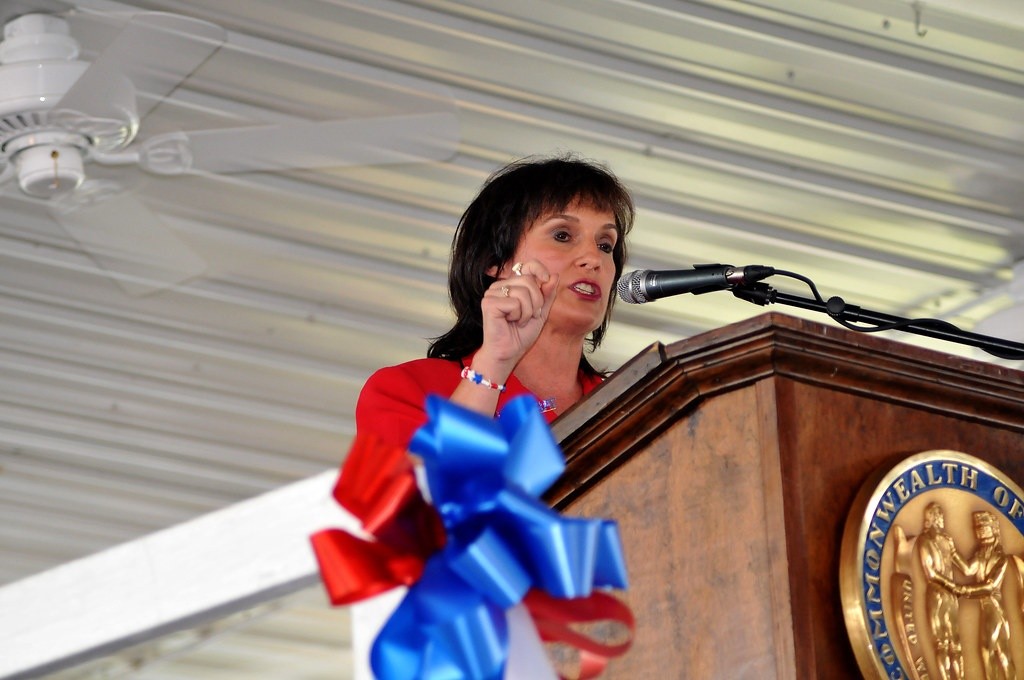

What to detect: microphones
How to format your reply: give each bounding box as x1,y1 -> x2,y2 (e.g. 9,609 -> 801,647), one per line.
618,265 -> 776,304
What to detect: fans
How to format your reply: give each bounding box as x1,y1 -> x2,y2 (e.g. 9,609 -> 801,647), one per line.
0,0 -> 467,297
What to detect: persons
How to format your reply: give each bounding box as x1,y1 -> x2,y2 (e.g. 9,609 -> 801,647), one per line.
355,152 -> 636,447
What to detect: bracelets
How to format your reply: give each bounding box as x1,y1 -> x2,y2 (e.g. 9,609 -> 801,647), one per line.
461,366 -> 506,391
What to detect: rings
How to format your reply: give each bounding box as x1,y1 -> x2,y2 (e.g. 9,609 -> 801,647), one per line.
512,262 -> 522,276
502,284 -> 511,296
